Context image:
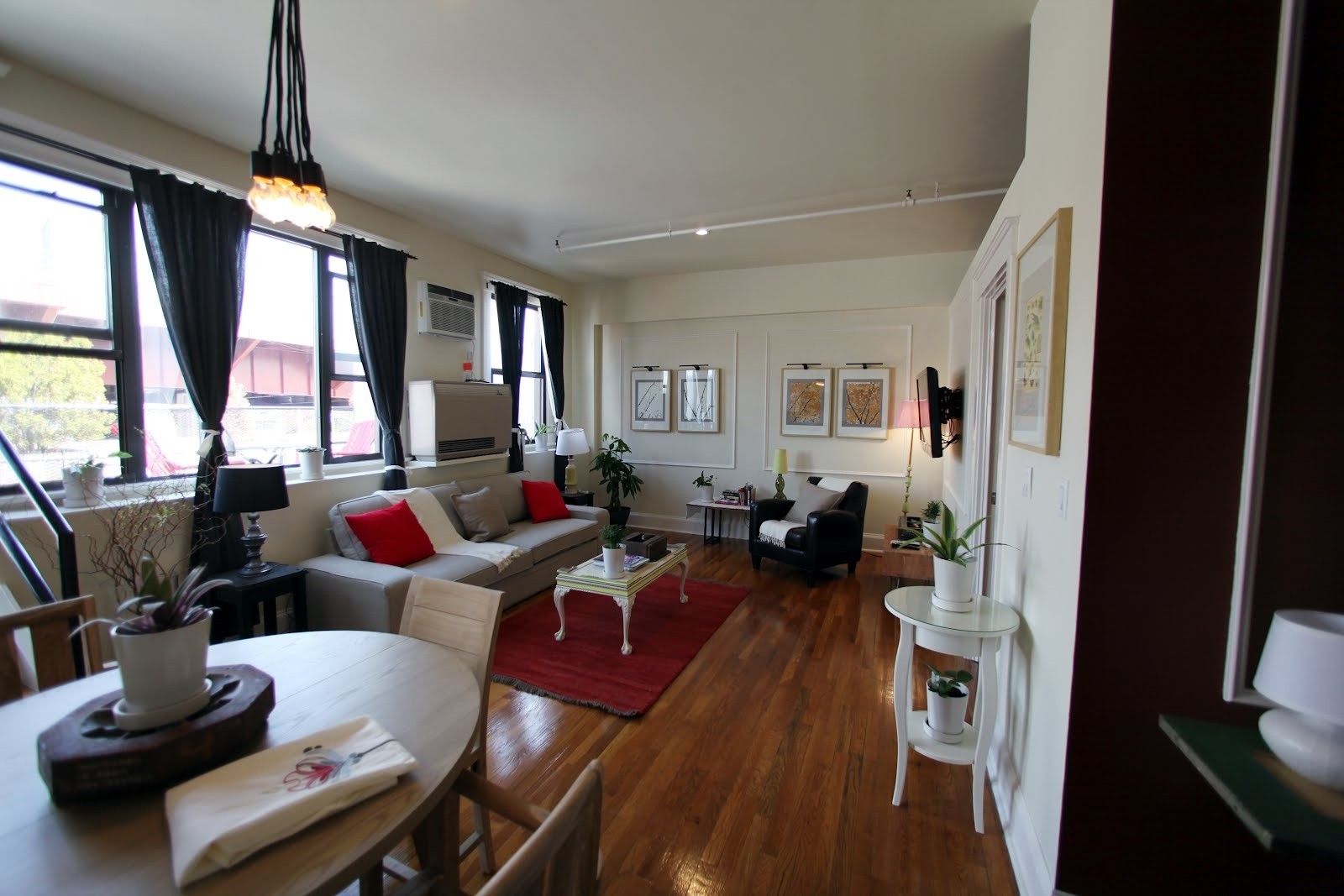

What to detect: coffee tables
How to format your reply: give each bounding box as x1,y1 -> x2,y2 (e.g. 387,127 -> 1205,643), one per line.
554,543 -> 690,654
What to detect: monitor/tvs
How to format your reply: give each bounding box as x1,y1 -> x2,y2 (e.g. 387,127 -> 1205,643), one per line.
914,367 -> 961,459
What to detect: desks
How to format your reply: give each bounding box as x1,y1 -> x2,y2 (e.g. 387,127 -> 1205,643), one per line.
0,630 -> 482,896
884,586 -> 1019,834
884,523 -> 936,590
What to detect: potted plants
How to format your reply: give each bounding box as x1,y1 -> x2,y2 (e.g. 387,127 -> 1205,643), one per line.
60,447 -> 327,730
893,501 -> 1022,744
534,421 -> 714,579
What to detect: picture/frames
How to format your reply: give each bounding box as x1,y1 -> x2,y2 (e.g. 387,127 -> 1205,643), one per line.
1007,208 -> 1073,456
629,368 -> 891,440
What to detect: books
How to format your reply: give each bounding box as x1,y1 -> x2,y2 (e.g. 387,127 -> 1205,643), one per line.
739,484 -> 757,506
593,555 -> 650,572
715,489 -> 739,505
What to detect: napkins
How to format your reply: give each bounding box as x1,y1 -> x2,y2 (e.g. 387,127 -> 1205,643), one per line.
165,712 -> 416,887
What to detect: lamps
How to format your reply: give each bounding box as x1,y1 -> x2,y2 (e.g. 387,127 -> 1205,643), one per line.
212,465 -> 290,578
893,400 -> 922,540
1253,608 -> 1344,791
632,363 -> 883,371
556,428 -> 590,494
772,449 -> 787,500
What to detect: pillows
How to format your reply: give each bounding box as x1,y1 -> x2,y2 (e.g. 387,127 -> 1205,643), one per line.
345,480 -> 571,567
784,482 -> 845,525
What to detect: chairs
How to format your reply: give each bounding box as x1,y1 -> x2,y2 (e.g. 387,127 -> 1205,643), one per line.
0,574 -> 605,895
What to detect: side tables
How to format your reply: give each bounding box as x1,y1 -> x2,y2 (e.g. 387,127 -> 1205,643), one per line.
562,491 -> 595,506
686,496 -> 750,545
208,557 -> 311,640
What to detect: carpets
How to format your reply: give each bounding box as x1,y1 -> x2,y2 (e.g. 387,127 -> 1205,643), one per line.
491,576 -> 751,717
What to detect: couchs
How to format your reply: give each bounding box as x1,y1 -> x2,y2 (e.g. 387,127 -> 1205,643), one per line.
748,476 -> 868,587
297,470 -> 611,635
